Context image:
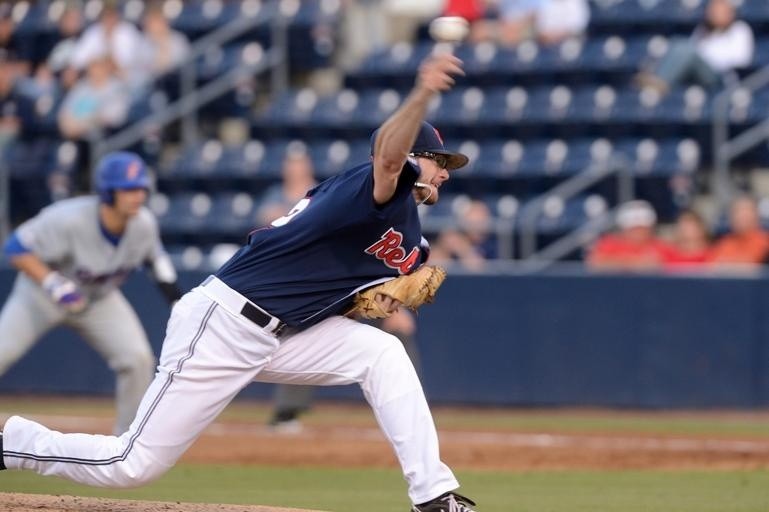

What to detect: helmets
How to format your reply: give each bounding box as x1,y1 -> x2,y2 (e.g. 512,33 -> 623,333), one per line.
93,150 -> 152,205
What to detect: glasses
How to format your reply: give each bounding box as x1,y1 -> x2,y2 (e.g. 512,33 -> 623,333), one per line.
409,152 -> 447,169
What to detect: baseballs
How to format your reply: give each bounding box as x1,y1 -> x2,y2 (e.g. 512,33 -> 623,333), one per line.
430,15 -> 468,42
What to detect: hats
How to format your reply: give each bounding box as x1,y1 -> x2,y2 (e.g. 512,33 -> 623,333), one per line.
369,121 -> 469,170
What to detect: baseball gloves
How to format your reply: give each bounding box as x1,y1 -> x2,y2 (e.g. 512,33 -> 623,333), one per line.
341,266 -> 446,321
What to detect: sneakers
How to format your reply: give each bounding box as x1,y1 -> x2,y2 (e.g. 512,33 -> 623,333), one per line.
410,492 -> 476,512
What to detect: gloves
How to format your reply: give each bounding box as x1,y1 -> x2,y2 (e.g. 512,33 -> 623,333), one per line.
40,270 -> 87,313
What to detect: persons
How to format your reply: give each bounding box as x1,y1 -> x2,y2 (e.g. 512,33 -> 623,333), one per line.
631,0 -> 758,101
0,0 -> 190,195
583,191 -> 769,269
379,0 -> 591,46
0,50 -> 475,512
254,139 -> 316,435
0,152 -> 184,438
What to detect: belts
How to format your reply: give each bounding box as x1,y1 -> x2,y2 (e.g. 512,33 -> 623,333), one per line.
240,301 -> 288,338
